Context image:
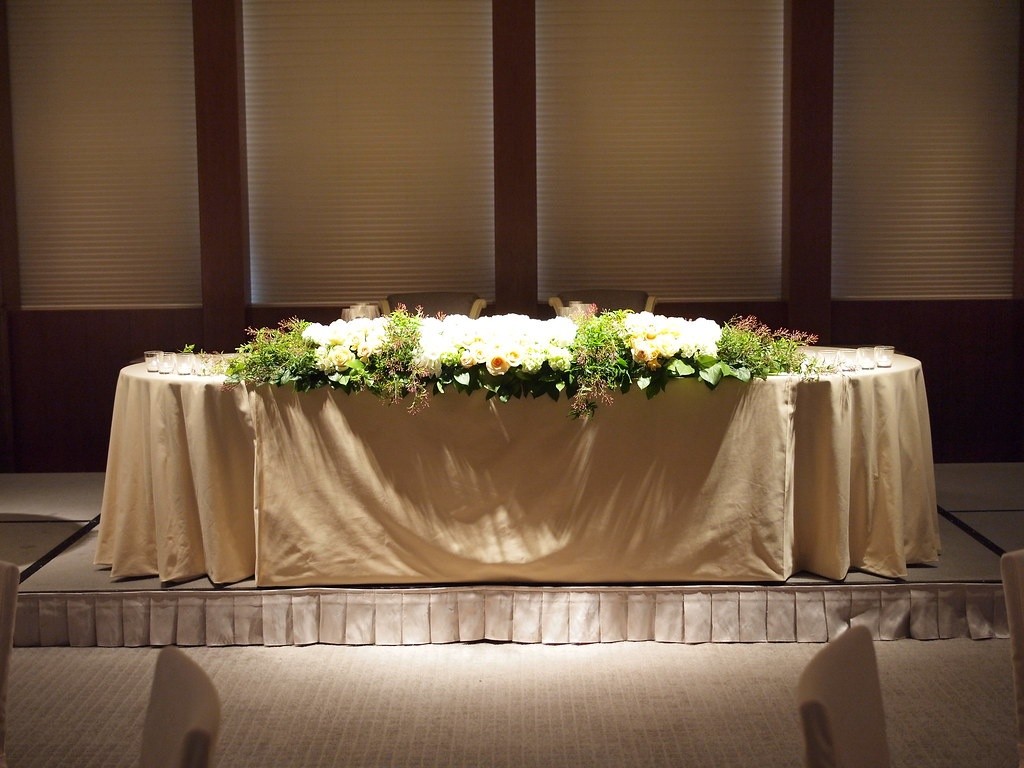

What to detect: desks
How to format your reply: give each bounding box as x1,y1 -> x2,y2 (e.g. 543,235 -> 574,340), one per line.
94,346 -> 941,583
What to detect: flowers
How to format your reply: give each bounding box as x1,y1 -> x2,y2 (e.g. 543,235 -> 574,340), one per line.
221,299 -> 851,420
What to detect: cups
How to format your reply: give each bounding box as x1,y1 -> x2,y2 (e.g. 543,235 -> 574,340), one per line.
339,303 -> 380,322
557,300 -> 593,318
800,346 -> 894,375
141,351 -> 224,376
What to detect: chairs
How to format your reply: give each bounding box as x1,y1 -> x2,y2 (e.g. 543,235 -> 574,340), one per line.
377,291 -> 488,319
549,287 -> 658,318
